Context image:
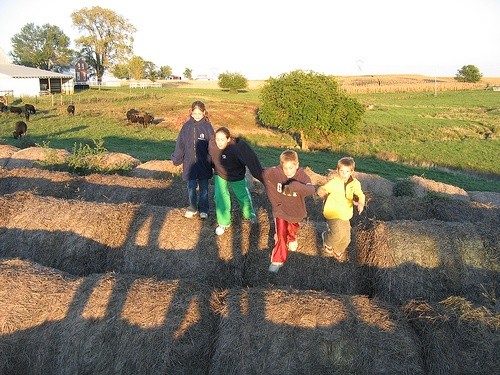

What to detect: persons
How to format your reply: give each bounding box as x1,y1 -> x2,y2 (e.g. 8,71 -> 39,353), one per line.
260,149 -> 316,273
208,126 -> 260,236
317,157 -> 366,260
173,101 -> 216,219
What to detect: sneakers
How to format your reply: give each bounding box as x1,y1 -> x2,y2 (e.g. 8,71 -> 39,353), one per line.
185,211 -> 198,218
200,212 -> 209,219
289,240 -> 299,251
215,224 -> 231,235
333,250 -> 344,260
321,231 -> 334,253
269,260 -> 284,273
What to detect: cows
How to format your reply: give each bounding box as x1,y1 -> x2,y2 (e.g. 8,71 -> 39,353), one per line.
13,121 -> 27,139
126,109 -> 153,128
67,105 -> 75,115
0,102 -> 36,121
